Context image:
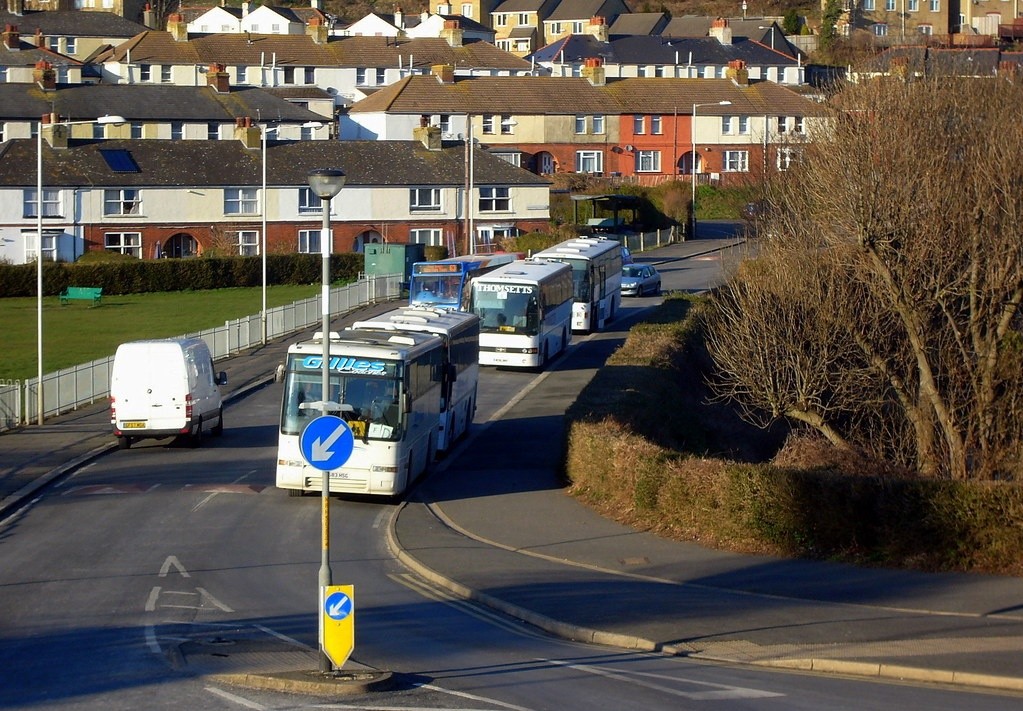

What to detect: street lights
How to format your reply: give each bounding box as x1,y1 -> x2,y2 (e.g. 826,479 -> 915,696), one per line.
468,120 -> 519,255
691,101 -> 733,242
306,167 -> 348,678
35,115 -> 128,428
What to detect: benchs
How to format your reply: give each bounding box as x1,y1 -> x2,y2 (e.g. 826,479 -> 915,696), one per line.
57,286 -> 103,307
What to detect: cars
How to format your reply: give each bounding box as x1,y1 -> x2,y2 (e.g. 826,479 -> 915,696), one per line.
621,262 -> 662,298
620,246 -> 633,265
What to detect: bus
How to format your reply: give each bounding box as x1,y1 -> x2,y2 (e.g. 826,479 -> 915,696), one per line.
274,305 -> 482,499
468,257 -> 574,371
532,237 -> 624,337
405,252 -> 525,313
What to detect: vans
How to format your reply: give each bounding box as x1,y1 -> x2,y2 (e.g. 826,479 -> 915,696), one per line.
107,334 -> 229,451
